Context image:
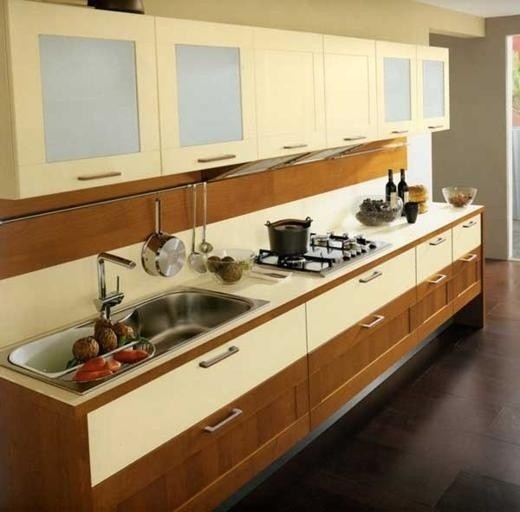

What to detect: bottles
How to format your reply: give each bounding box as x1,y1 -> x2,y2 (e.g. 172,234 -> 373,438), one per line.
386,169 -> 397,201
398,168 -> 409,204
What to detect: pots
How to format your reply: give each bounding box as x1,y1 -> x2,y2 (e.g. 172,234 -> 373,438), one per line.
141,199 -> 186,279
264,216 -> 313,256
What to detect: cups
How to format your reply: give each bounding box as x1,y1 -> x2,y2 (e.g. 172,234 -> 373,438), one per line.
403,202 -> 419,223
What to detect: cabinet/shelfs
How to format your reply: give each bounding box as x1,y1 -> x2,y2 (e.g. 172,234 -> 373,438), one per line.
325,34 -> 377,151
304,244 -> 417,436
417,227 -> 452,349
0,301 -> 313,511
453,207 -> 484,315
417,44 -> 451,135
0,0 -> 162,203
376,40 -> 417,141
254,26 -> 324,162
155,16 -> 257,181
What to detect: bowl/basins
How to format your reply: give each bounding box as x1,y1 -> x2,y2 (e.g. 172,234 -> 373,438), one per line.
203,248 -> 256,287
351,195 -> 403,228
440,186 -> 480,210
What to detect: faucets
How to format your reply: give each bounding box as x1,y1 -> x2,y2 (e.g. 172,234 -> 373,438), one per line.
93,252 -> 136,319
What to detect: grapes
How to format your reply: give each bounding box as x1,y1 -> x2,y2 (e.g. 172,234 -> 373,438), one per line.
356,198 -> 397,225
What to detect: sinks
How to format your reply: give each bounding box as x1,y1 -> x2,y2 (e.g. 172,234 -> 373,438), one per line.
1,283 -> 270,396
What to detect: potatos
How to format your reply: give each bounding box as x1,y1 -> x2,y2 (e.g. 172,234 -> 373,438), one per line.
208,256 -> 242,281
72,320 -> 149,381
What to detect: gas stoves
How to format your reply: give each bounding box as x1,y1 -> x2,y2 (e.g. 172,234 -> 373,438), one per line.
252,230 -> 392,278
